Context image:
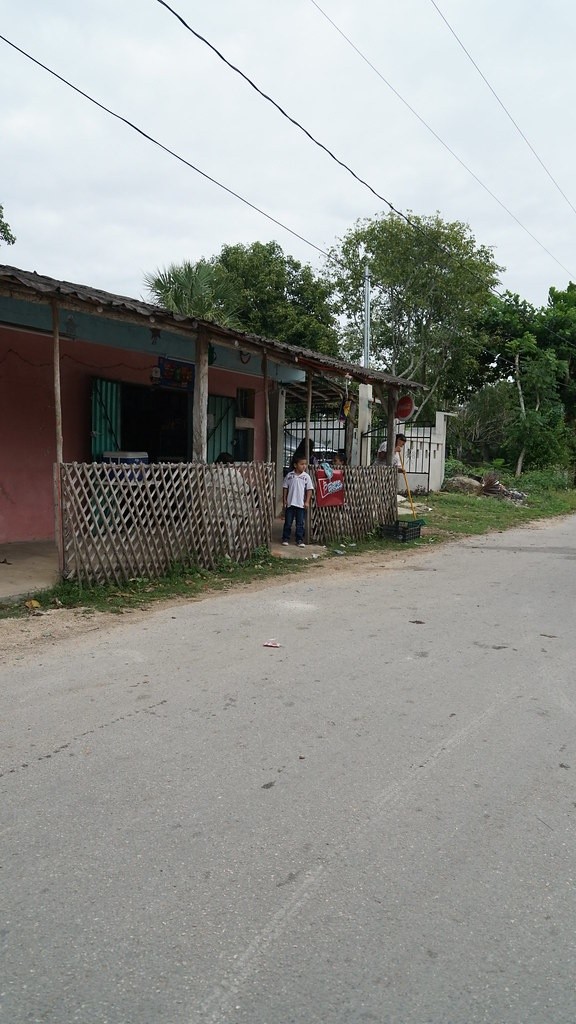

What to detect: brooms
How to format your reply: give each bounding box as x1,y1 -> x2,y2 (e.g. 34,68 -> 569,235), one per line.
395,448 -> 427,529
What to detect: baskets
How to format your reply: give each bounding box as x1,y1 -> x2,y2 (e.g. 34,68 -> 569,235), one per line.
381,519 -> 422,543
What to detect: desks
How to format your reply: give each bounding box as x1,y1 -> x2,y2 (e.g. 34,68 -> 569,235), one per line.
94,480 -> 161,541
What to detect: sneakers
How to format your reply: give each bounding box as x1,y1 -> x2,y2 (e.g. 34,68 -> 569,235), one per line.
282,536 -> 290,545
297,540 -> 305,547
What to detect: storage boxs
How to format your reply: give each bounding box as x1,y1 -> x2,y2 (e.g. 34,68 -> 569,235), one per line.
103,451 -> 148,481
381,520 -> 422,543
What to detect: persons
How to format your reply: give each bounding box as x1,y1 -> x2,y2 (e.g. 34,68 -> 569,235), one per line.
205,452 -> 250,552
371,433 -> 405,526
331,454 -> 346,466
281,438 -> 315,548
